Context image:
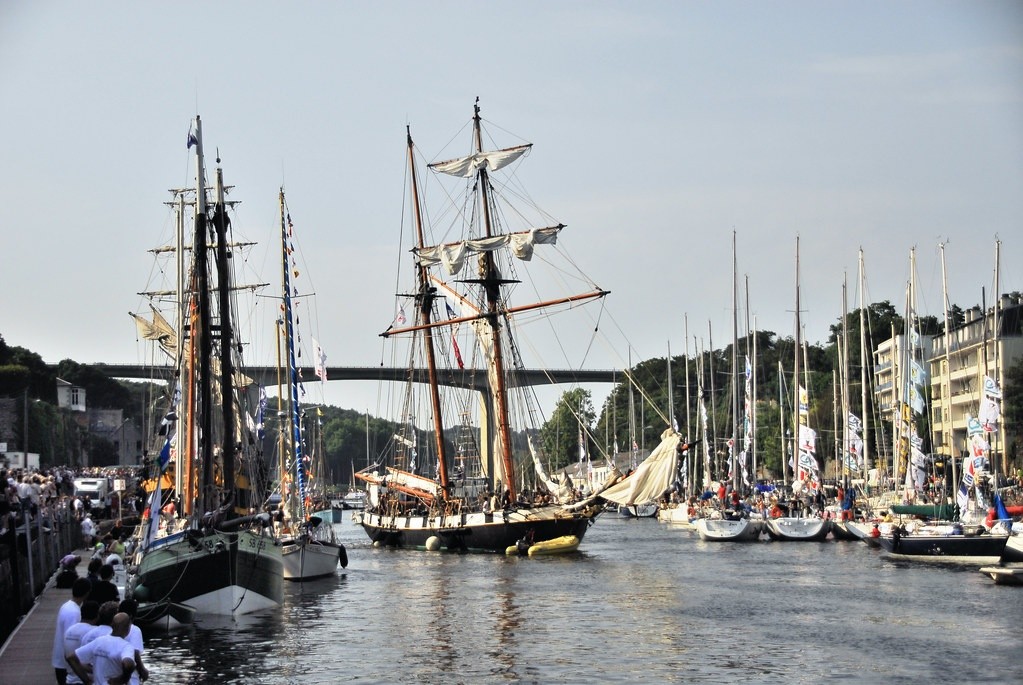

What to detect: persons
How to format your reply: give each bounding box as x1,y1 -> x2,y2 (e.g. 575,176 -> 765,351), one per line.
483,485 -> 550,513
688,478 -> 854,520
0,454 -> 149,685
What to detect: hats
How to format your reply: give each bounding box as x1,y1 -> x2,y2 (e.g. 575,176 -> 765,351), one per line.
86,512 -> 92,517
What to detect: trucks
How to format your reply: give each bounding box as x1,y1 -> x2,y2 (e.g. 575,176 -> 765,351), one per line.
72,476 -> 112,519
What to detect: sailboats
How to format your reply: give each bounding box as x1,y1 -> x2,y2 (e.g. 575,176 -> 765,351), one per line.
351,97 -> 636,558
342,457 -> 368,509
59,114 -> 347,641
542,228 -> 1023,589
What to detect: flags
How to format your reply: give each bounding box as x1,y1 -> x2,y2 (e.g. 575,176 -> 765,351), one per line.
957,378 -> 1004,507
157,441 -> 172,471
901,329 -> 927,494
312,338 -> 327,381
845,412 -> 863,474
445,303 -> 461,336
396,310 -> 406,324
797,385 -> 820,472
453,338 -> 464,369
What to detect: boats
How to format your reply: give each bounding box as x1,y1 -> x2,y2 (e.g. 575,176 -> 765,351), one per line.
504,533 -> 580,557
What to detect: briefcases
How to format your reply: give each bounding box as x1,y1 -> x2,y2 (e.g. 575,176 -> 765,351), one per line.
52,570 -> 79,589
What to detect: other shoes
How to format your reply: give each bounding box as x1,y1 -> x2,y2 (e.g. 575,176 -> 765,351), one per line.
86,548 -> 90,551
